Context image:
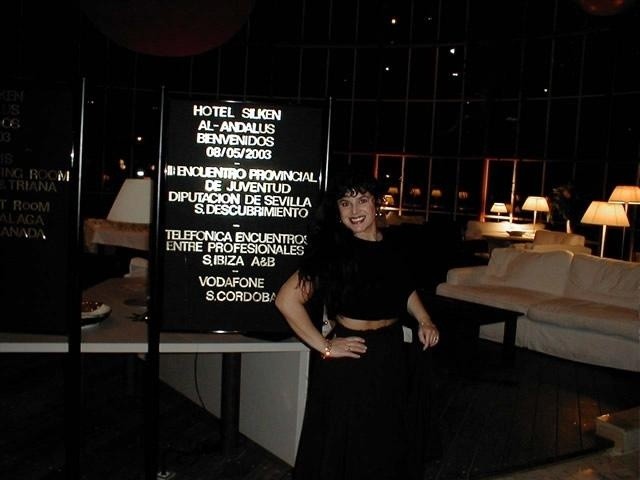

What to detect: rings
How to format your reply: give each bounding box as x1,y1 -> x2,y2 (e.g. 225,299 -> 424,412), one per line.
345,346 -> 350,351
434,337 -> 437,341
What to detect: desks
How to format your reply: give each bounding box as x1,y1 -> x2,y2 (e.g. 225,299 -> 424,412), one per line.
482,231 -> 535,255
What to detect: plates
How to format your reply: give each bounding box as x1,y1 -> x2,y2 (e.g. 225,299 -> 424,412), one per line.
506,231 -> 526,236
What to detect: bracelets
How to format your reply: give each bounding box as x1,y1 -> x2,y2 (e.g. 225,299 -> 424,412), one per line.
320,338 -> 332,360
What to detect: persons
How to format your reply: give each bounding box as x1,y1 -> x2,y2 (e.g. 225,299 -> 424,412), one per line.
273,159 -> 452,479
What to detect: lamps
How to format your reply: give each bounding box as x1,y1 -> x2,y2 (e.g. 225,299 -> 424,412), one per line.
579,184 -> 640,260
522,196 -> 550,227
104,175 -> 156,228
490,203 -> 508,222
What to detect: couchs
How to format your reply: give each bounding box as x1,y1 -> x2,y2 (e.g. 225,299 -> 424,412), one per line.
438,250 -> 639,377
